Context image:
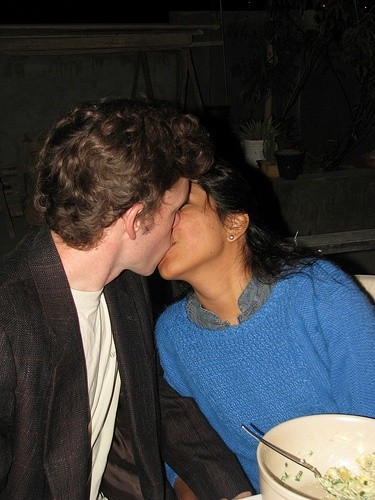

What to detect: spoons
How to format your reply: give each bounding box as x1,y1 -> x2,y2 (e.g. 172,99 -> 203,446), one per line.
242,426 -> 349,499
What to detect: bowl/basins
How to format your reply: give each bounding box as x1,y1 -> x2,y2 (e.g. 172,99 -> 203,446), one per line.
257,414 -> 375,500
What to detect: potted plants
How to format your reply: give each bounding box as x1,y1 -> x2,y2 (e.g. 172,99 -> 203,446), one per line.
232,114 -> 282,168
256,137 -> 288,181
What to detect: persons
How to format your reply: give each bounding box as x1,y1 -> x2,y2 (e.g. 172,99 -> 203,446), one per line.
157,139 -> 375,500
0,90 -> 257,500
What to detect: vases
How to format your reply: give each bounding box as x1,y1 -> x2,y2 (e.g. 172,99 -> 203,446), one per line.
274,148 -> 307,180
